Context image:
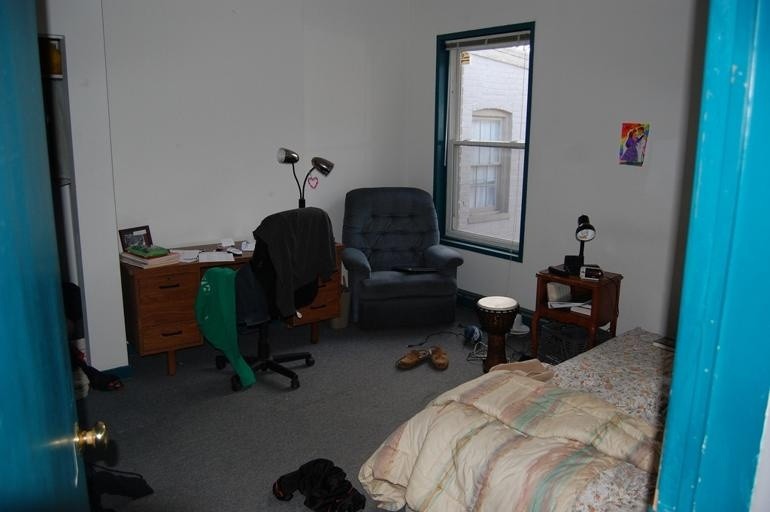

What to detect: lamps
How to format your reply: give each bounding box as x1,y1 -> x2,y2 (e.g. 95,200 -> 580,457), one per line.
277,146 -> 334,209
569,213 -> 596,275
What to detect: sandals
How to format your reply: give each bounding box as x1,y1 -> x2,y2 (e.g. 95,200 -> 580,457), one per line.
395,342 -> 431,369
429,342 -> 449,371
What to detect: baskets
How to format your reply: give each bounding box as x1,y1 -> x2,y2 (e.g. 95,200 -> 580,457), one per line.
538,321 -> 613,365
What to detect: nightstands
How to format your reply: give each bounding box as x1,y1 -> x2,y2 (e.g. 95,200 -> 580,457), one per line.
532,262 -> 623,360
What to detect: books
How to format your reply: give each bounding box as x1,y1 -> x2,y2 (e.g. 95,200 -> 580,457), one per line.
548,293 -> 592,308
120,252 -> 182,269
581,303 -> 592,308
571,306 -> 591,316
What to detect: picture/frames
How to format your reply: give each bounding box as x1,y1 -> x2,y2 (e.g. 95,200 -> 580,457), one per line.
119,225 -> 152,255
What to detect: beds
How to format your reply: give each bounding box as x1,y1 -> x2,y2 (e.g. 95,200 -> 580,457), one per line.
376,324 -> 676,511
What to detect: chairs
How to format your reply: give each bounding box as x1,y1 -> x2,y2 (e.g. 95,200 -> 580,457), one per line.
342,186 -> 464,328
195,208 -> 336,392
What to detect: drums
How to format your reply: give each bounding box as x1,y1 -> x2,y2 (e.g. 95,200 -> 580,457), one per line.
476,296 -> 520,374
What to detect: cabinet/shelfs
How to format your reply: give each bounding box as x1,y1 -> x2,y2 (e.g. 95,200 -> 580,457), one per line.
120,238 -> 348,376
